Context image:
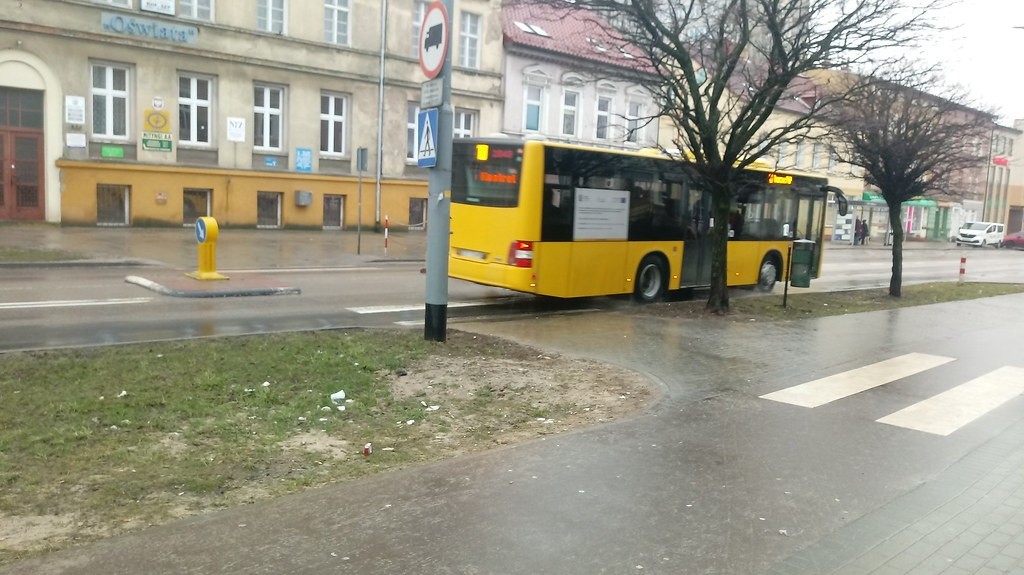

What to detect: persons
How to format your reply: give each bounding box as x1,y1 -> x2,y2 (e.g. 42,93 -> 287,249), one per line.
859,219 -> 869,245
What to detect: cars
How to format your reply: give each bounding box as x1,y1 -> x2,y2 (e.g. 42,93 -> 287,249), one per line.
1004,229 -> 1024,249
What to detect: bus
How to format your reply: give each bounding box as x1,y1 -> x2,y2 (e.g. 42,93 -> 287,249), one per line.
451,137 -> 848,303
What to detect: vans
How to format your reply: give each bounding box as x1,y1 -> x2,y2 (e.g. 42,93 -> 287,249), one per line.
956,222 -> 1006,250
